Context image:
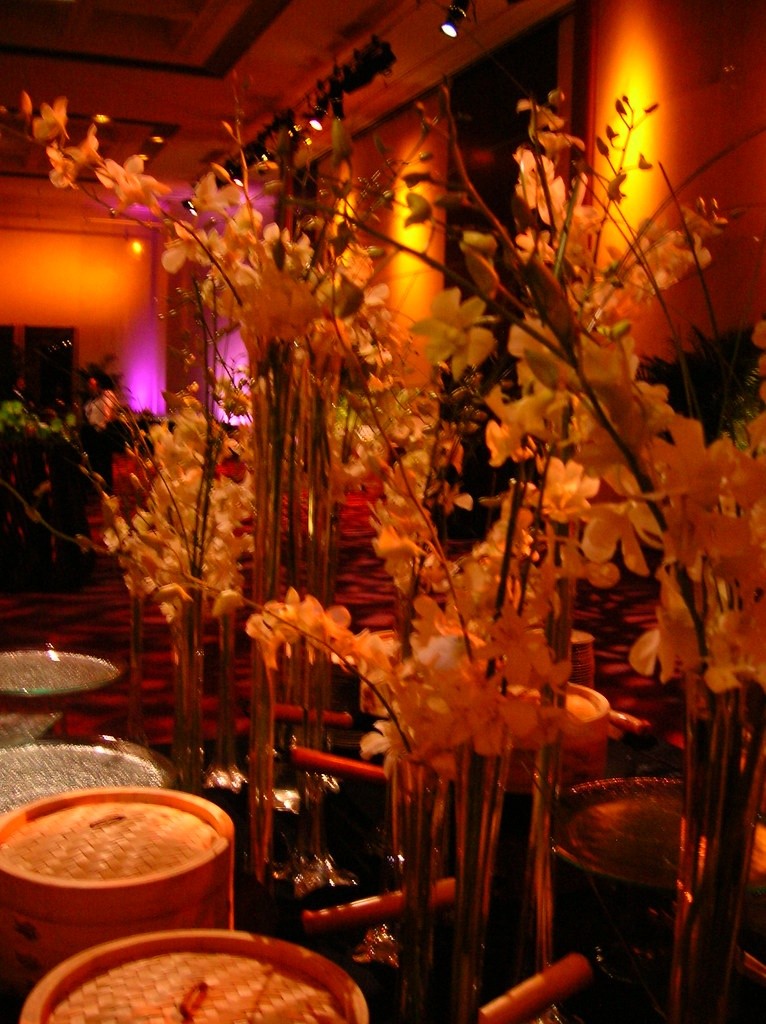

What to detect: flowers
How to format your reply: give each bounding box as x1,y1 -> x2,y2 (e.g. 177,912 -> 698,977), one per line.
0,42 -> 766,780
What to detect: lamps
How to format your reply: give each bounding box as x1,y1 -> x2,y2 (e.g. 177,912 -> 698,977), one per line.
440,0 -> 470,38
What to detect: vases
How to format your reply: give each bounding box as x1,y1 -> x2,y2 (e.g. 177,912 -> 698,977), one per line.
122,336 -> 766,1024
